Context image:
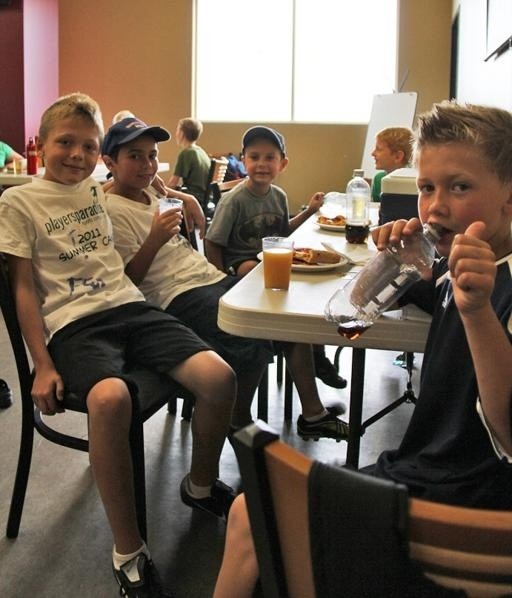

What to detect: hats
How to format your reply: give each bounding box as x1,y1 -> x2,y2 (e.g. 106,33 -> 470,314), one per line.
242,126 -> 285,158
101,118 -> 170,157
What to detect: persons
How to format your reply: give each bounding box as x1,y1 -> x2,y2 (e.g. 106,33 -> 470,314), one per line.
0,91 -> 238,598
370,126 -> 417,202
97,109 -> 366,466
214,99 -> 512,598
0,141 -> 25,172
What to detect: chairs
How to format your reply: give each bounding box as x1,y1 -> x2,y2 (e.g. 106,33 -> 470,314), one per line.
1,157 -> 295,563
231,418 -> 512,597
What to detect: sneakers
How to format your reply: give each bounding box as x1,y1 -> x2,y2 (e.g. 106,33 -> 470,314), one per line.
0,379 -> 12,408
297,415 -> 349,442
314,353 -> 346,388
112,552 -> 164,598
180,473 -> 236,522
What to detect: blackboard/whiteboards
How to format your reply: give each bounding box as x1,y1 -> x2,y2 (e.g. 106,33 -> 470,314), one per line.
361,92 -> 417,179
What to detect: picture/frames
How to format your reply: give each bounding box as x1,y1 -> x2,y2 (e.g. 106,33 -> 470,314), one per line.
482,1 -> 512,61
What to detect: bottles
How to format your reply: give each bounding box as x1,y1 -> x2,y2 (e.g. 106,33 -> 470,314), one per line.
27,137 -> 37,174
324,221 -> 446,342
346,169 -> 370,244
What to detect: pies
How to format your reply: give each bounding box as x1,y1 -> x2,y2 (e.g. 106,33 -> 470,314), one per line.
292,247 -> 341,264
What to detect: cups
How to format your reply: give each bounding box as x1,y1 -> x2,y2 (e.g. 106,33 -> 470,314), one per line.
159,198 -> 183,218
262,237 -> 294,292
14,159 -> 22,174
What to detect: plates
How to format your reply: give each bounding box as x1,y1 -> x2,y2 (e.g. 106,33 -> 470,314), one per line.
257,249 -> 349,273
312,218 -> 345,232
